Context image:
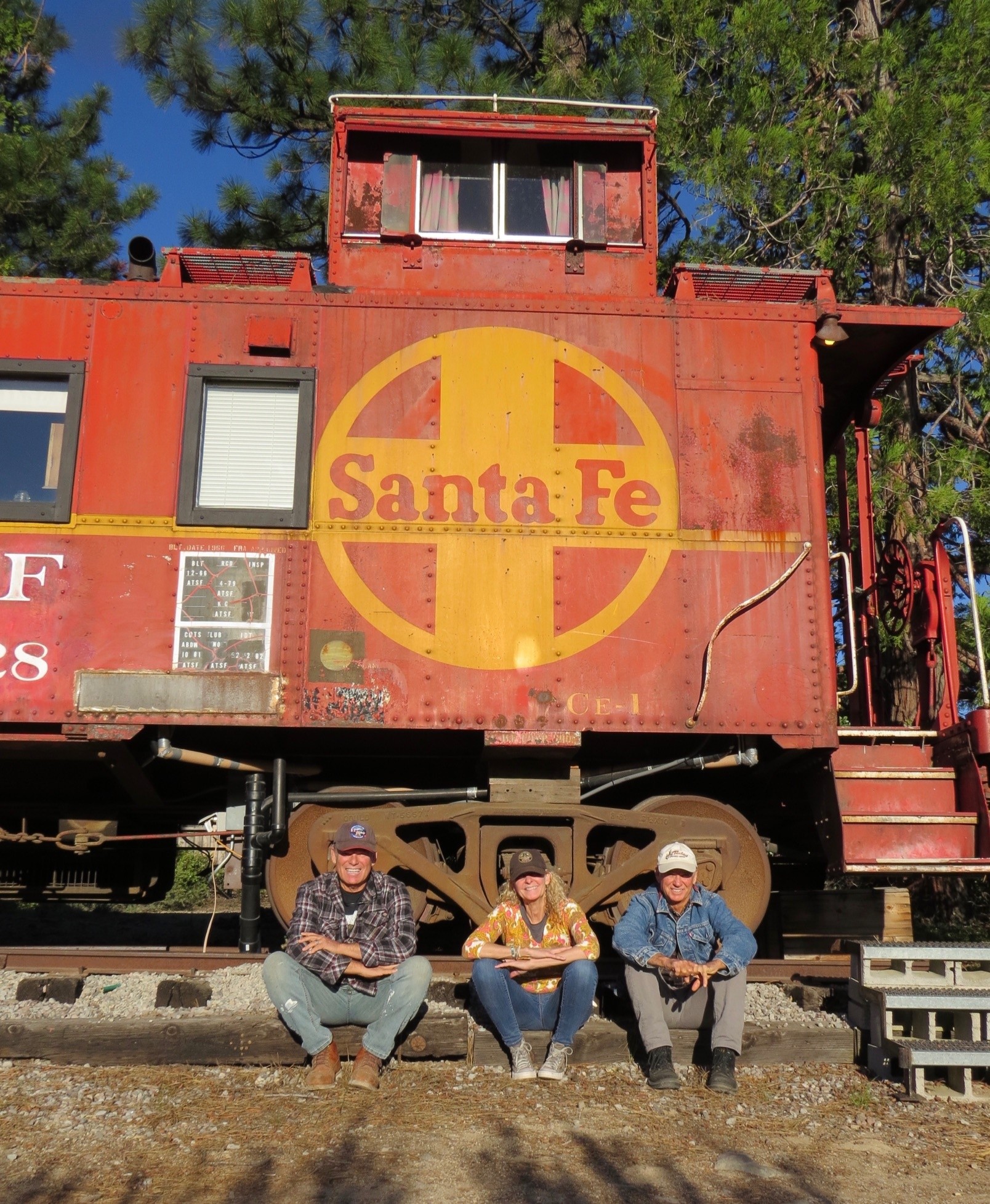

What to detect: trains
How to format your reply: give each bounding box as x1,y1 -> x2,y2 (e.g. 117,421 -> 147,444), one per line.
2,92 -> 990,951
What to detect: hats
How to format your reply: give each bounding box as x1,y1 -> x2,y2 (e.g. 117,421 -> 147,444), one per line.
333,821 -> 376,854
510,849 -> 547,882
657,842 -> 698,873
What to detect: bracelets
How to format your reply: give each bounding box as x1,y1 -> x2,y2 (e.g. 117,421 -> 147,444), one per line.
511,945 -> 521,959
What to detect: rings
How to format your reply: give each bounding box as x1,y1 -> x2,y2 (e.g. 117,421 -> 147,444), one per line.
317,941 -> 319,945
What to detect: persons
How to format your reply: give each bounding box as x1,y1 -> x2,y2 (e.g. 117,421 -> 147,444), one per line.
462,850 -> 600,1080
612,842 -> 758,1093
262,822 -> 432,1092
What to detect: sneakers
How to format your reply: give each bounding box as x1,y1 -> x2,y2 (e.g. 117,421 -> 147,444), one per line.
647,1045 -> 680,1089
510,1037 -> 537,1079
705,1047 -> 739,1094
538,1041 -> 573,1080
304,1037 -> 342,1091
347,1046 -> 382,1091
